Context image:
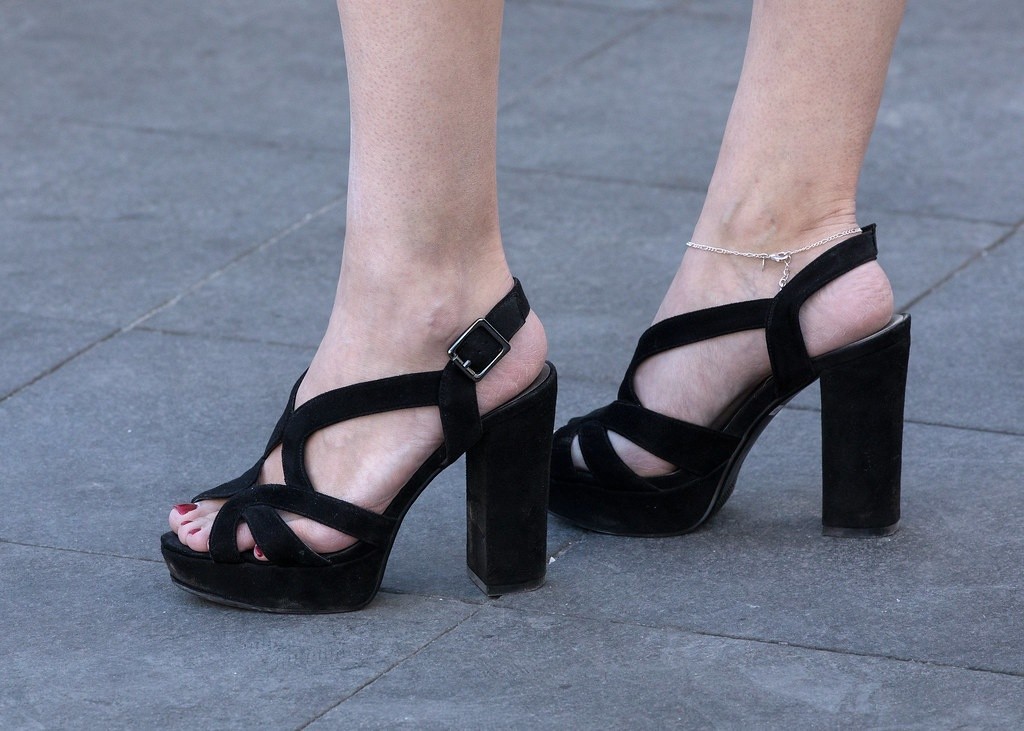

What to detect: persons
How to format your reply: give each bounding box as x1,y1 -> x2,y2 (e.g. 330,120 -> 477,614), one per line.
160,1 -> 911,616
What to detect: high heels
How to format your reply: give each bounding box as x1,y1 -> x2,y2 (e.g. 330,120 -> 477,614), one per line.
546,223 -> 914,537
159,278 -> 557,614
